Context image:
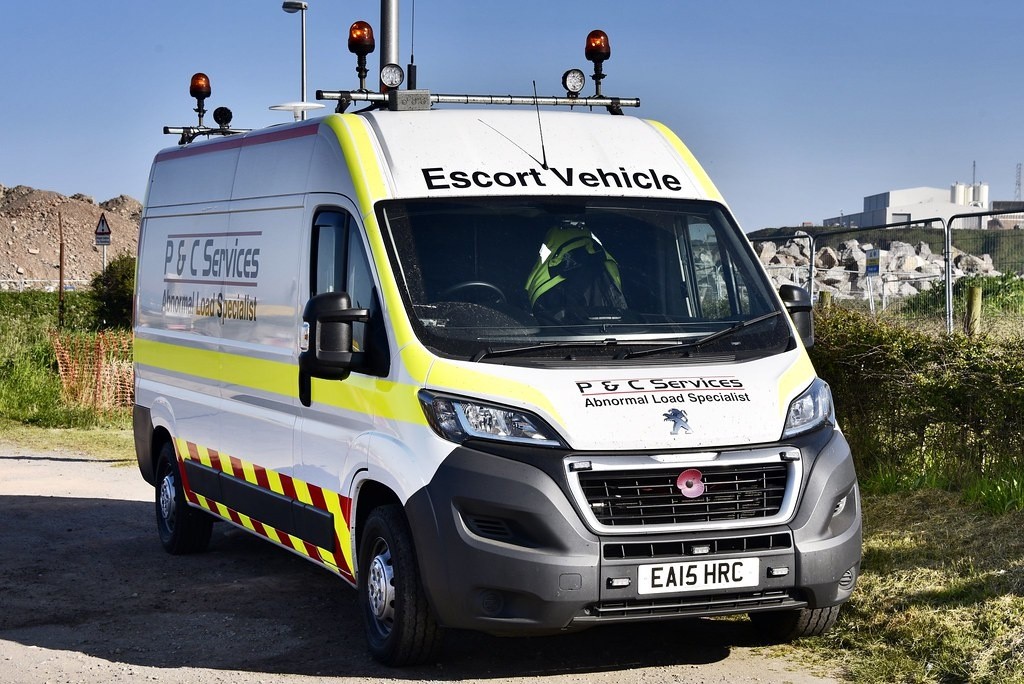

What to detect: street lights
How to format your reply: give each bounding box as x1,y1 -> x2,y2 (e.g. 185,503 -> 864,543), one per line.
280,0 -> 309,123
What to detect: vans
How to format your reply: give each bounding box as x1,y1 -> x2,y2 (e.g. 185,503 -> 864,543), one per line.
132,1 -> 865,671
